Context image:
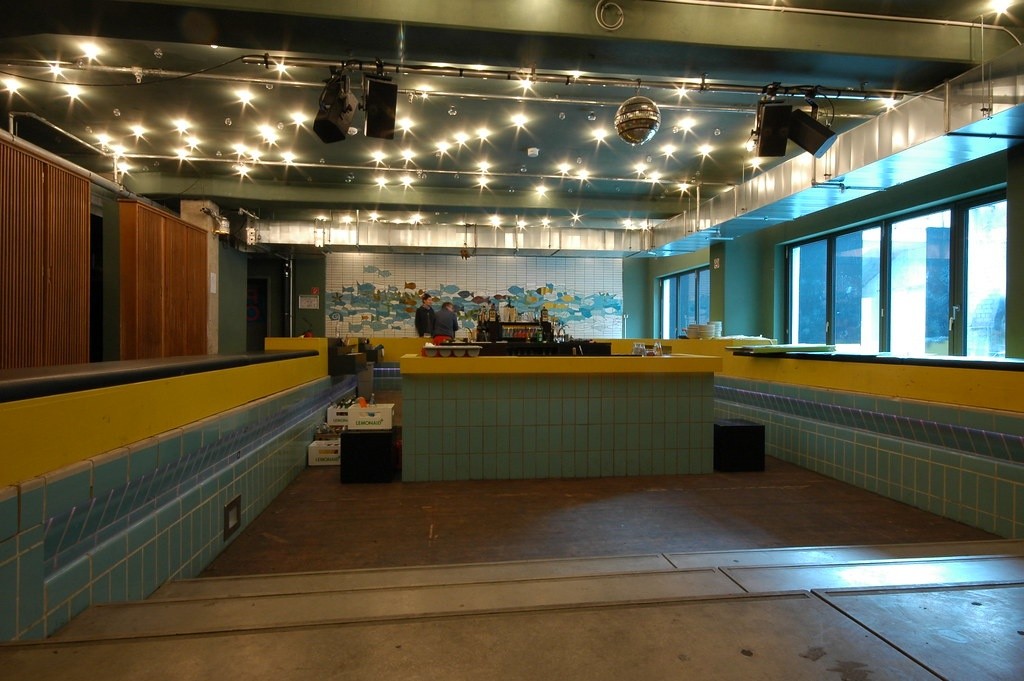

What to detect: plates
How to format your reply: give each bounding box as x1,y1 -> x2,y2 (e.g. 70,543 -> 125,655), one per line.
706,320 -> 722,338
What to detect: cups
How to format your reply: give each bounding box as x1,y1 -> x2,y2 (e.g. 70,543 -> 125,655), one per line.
631,343 -> 663,355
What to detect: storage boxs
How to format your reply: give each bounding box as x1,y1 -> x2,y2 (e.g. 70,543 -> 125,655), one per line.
307,426 -> 342,465
308,402 -> 394,429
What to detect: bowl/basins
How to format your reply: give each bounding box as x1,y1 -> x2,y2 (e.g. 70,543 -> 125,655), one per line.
686,324 -> 716,339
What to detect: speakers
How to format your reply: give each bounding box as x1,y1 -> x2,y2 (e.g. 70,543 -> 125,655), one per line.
314,85 -> 358,143
364,79 -> 398,140
756,103 -> 792,157
788,109 -> 837,158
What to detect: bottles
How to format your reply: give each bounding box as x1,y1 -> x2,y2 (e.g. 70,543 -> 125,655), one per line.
541,306 -> 548,322
509,306 -> 516,323
477,303 -> 496,326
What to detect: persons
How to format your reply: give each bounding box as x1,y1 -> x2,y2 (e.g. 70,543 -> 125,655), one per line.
431,301 -> 459,339
415,293 -> 436,337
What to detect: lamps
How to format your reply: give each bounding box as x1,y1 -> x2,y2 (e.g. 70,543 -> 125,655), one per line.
311,66 -> 398,145
753,89 -> 837,160
